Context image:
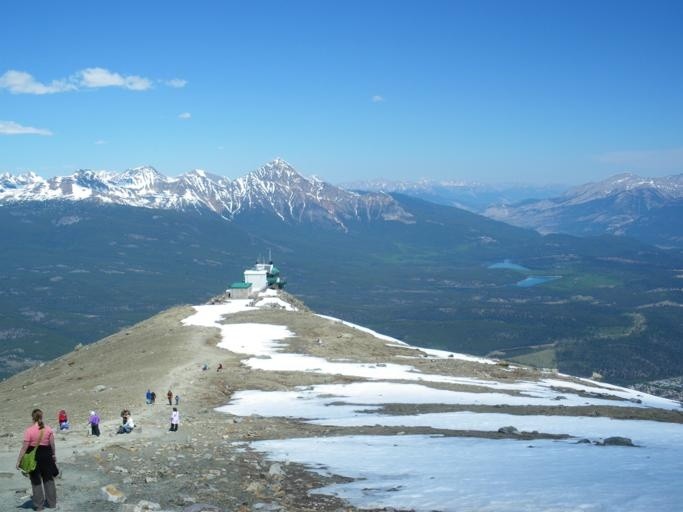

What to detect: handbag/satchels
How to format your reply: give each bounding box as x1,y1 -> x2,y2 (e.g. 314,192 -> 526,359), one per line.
19,451 -> 37,473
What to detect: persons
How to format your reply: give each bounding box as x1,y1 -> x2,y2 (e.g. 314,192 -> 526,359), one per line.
166,389 -> 172,405
145,388 -> 156,405
116,407 -> 133,434
83,409 -> 100,436
15,408 -> 59,511
167,407 -> 178,432
57,409 -> 69,440
